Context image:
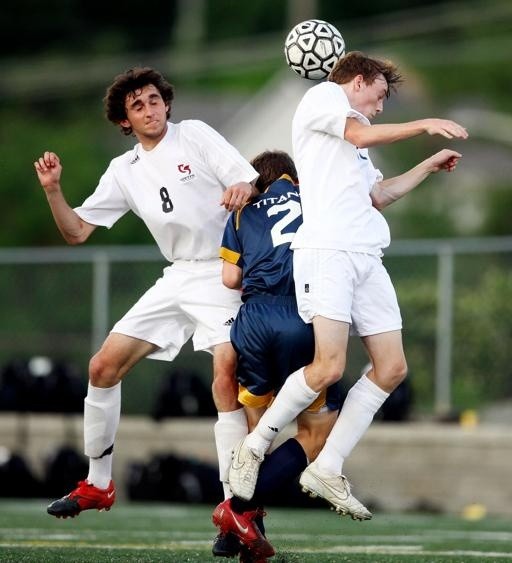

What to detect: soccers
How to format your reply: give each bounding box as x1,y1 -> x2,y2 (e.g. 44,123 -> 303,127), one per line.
282,18 -> 346,80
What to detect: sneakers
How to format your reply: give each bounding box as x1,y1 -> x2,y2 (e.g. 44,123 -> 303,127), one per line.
298,462 -> 373,522
227,434 -> 265,502
211,497 -> 276,563
47,480 -> 115,519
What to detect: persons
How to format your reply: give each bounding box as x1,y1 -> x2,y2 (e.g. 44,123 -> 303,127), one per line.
34,68 -> 260,558
227,52 -> 469,522
212,150 -> 342,562
